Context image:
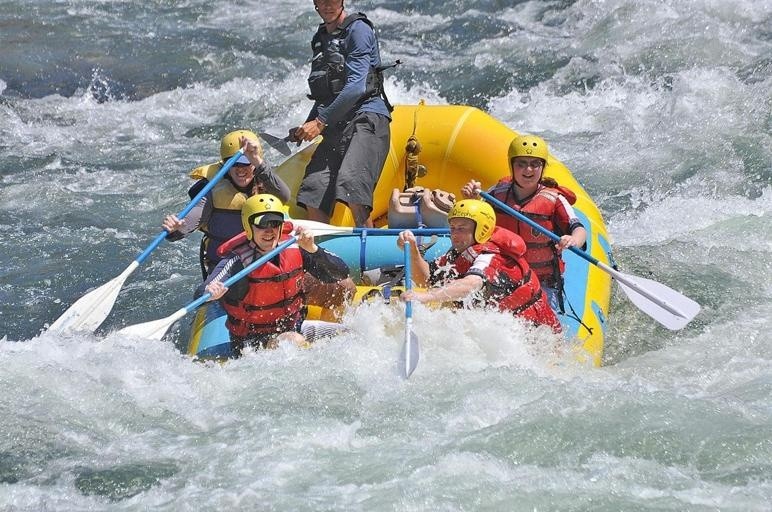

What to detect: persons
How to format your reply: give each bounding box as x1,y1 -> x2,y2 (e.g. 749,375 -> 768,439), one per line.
192,193 -> 350,355
161,130 -> 290,282
396,198 -> 563,353
461,134 -> 586,311
289,0 -> 403,228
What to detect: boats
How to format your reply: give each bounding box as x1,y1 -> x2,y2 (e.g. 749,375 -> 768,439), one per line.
185,104 -> 615,370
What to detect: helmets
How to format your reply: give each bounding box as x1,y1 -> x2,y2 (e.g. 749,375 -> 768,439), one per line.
447,199 -> 496,244
508,135 -> 548,177
220,130 -> 262,161
241,194 -> 283,240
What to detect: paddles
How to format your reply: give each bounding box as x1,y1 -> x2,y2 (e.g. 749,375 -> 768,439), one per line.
259,126 -> 299,158
46,148 -> 245,337
398,242 -> 419,378
474,187 -> 699,332
290,218 -> 457,238
108,235 -> 300,341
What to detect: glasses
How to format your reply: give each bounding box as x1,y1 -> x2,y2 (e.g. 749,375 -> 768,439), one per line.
250,213 -> 283,228
519,160 -> 541,167
